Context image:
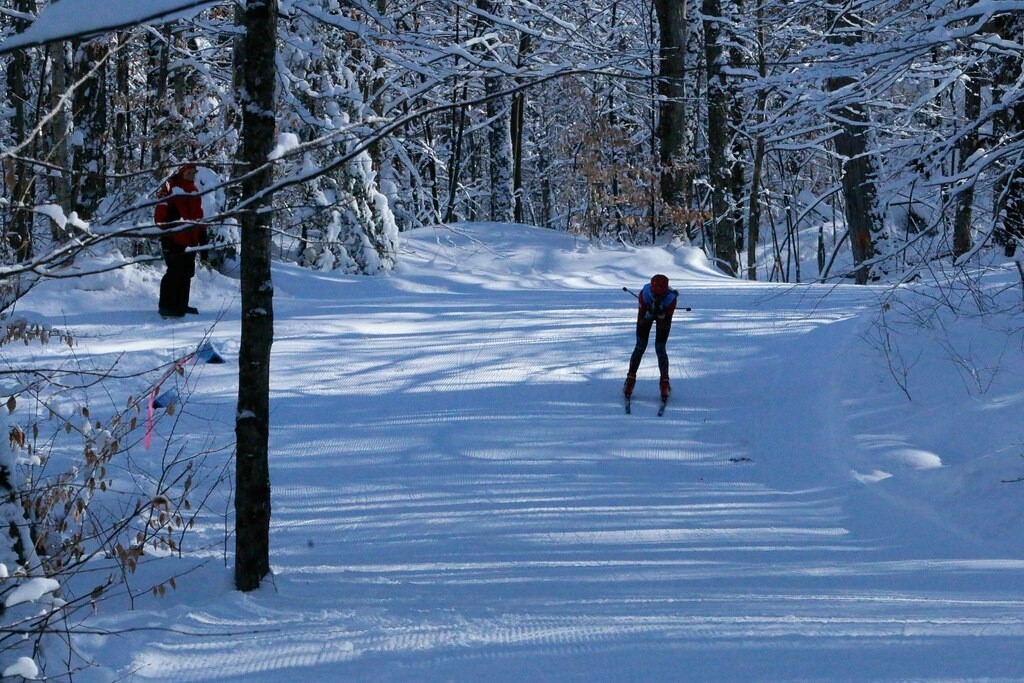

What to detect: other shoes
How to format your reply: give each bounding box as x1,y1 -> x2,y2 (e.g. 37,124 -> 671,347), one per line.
158,306 -> 186,318
180,303 -> 198,314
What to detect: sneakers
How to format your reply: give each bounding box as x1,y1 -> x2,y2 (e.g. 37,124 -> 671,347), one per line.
659,378 -> 670,396
623,372 -> 636,393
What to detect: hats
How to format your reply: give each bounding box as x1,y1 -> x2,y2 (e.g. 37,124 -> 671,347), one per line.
650,274 -> 669,292
178,159 -> 197,175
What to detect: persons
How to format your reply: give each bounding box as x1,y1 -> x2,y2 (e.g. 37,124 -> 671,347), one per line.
153,163 -> 210,318
622,275 -> 678,404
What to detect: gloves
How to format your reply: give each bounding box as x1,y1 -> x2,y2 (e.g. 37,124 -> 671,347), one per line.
199,232 -> 210,244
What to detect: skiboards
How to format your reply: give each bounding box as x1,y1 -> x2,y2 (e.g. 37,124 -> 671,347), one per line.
624,386 -> 671,416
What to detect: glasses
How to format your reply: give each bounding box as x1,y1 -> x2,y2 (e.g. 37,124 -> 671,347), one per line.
652,293 -> 669,300
